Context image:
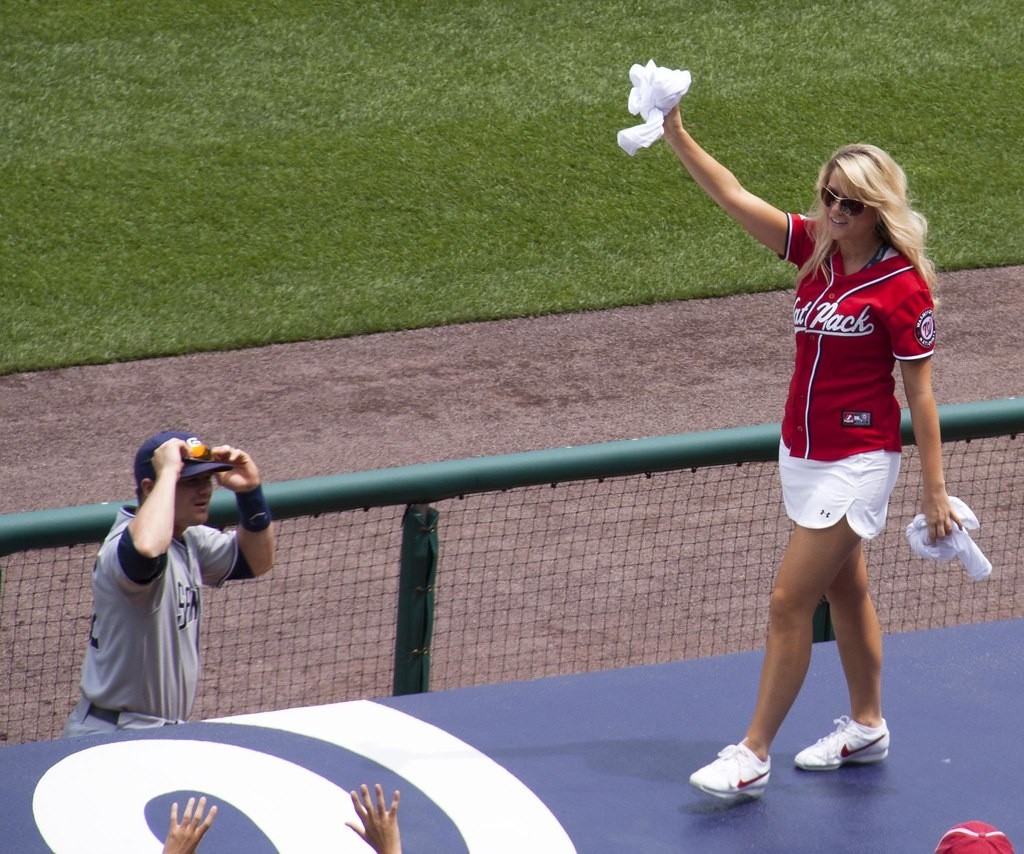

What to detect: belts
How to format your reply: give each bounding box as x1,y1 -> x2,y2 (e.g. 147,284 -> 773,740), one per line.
88,703 -> 176,726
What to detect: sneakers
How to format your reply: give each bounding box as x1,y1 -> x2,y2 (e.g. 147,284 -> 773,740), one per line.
795,715 -> 890,771
689,737 -> 771,798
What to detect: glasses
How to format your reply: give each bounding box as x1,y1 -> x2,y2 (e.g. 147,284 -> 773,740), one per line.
139,445 -> 224,468
820,186 -> 870,217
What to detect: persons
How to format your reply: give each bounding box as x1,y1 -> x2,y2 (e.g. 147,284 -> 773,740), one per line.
163,783 -> 403,854
57,428 -> 277,737
935,820 -> 1013,854
660,104 -> 964,796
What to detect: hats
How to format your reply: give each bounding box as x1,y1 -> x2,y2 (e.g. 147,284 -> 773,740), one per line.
134,431 -> 234,489
934,820 -> 1015,854
